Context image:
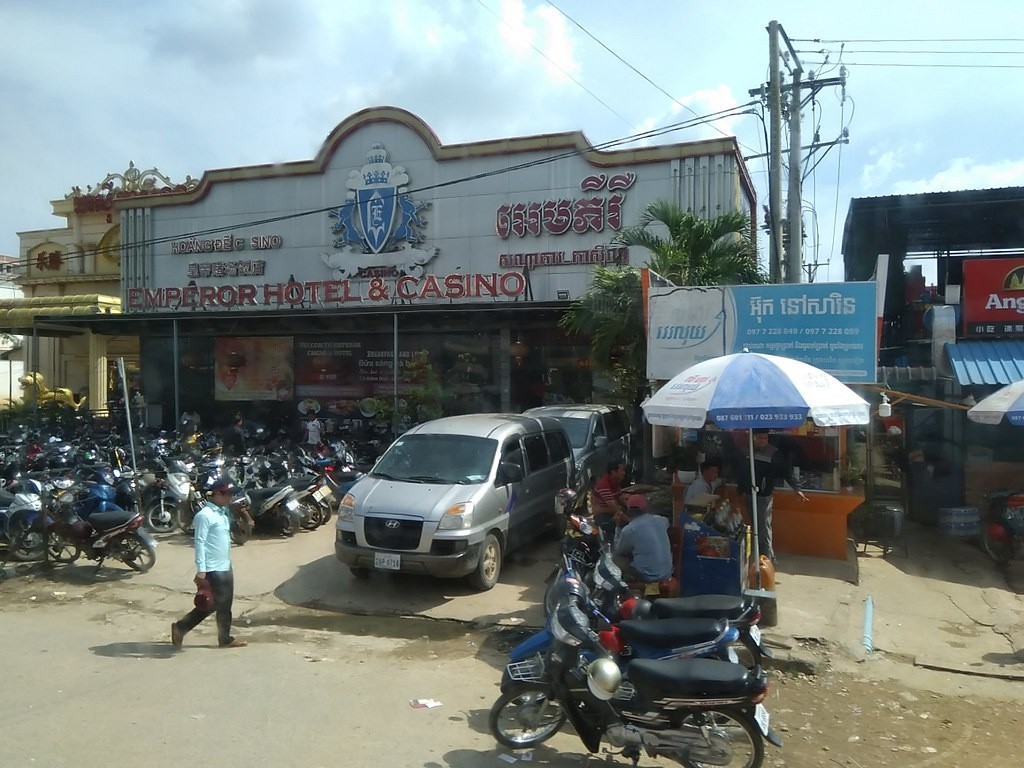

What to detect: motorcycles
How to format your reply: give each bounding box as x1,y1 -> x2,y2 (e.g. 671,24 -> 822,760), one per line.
978,487 -> 1023,565
488,486 -> 783,768
1,412 -> 391,578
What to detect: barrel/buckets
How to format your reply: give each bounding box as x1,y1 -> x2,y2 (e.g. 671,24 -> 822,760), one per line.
748,555 -> 775,592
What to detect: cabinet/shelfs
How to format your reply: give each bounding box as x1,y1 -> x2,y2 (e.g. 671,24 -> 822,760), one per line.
680,492 -> 751,596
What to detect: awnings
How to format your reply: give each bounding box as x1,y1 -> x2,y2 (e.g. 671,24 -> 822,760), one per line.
944,340 -> 1024,386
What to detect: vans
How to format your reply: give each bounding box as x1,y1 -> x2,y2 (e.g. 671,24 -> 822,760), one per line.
521,403 -> 634,514
334,412 -> 577,593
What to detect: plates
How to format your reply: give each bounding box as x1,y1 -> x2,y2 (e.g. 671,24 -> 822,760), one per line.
298,402 -> 320,414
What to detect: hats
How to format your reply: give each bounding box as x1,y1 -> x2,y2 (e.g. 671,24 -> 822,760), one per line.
212,480 -> 243,495
626,494 -> 647,510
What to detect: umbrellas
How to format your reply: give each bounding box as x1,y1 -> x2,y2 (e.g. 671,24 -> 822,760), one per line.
966,381 -> 1024,426
642,352 -> 870,590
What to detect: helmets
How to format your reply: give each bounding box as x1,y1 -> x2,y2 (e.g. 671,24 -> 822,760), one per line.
587,658 -> 622,700
620,598 -> 654,621
598,625 -> 624,653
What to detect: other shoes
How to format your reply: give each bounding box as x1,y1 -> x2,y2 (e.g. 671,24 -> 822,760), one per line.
172,623 -> 183,650
220,640 -> 247,648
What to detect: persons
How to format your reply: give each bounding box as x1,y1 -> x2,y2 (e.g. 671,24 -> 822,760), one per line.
592,460 -> 628,541
735,428 -> 810,565
681,460 -> 725,508
613,494 -> 675,589
302,410 -> 325,444
171,477 -> 248,649
180,406 -> 201,426
222,416 -> 246,456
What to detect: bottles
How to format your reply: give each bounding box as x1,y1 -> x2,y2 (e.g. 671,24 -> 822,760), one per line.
937,506 -> 979,536
715,498 -> 742,535
